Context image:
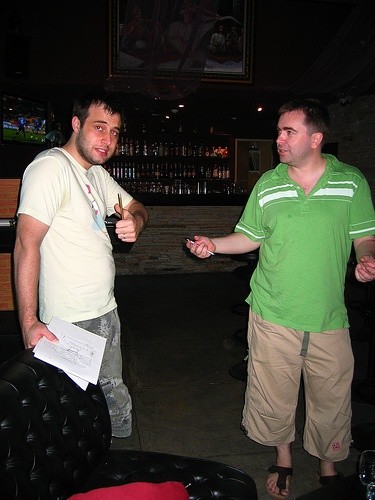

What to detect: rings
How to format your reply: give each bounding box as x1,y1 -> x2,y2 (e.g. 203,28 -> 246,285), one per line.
123,234 -> 125,239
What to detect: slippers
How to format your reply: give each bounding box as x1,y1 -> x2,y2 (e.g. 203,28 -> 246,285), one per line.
315,469 -> 343,486
265,464 -> 293,499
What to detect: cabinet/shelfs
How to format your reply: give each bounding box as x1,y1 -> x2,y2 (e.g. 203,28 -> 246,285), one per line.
103,139 -> 273,201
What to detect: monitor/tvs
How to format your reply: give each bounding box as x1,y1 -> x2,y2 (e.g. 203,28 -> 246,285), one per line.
0,91 -> 50,145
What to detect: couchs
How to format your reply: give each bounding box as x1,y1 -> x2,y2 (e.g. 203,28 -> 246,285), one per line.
0,351 -> 258,500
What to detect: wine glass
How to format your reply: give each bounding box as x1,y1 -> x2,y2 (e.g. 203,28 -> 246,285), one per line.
360,450 -> 375,500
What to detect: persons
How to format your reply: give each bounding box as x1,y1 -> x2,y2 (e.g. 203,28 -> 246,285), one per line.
16,113 -> 42,140
13,90 -> 149,437
185,97 -> 375,498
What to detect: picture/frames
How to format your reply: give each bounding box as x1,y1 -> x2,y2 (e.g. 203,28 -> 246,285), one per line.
108,0 -> 253,85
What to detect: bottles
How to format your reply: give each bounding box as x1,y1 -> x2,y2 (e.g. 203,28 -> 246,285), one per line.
102,120 -> 235,195
